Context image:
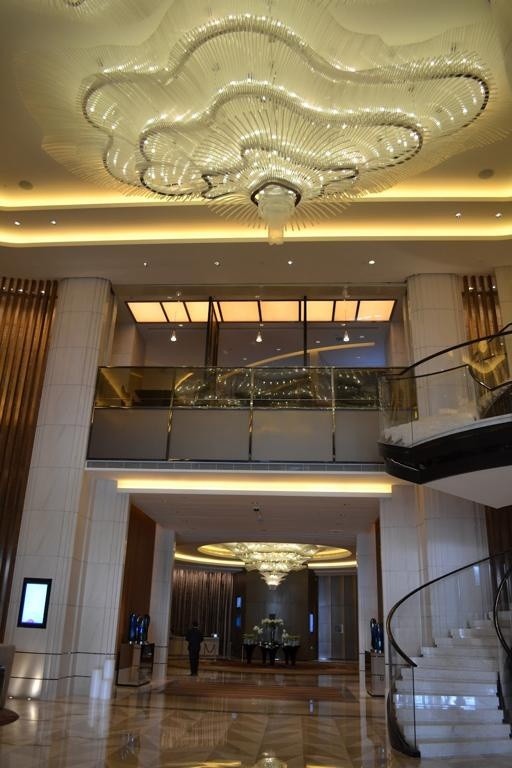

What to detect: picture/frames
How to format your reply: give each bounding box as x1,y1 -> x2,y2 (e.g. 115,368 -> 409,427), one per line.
17,578 -> 52,629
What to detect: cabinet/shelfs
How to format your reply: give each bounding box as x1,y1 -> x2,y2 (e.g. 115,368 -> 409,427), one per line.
169,636 -> 219,662
365,650 -> 392,696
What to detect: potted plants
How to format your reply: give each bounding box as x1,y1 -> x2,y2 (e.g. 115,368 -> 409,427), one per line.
243,613 -> 300,665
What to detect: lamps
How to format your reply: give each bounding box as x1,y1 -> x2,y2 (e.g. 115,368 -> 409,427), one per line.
81,14 -> 489,246
221,542 -> 327,591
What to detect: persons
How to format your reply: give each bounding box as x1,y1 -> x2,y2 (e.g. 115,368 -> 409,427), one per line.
185,620 -> 204,676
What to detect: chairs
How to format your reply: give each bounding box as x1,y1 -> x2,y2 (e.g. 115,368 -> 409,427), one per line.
0,644 -> 19,726
